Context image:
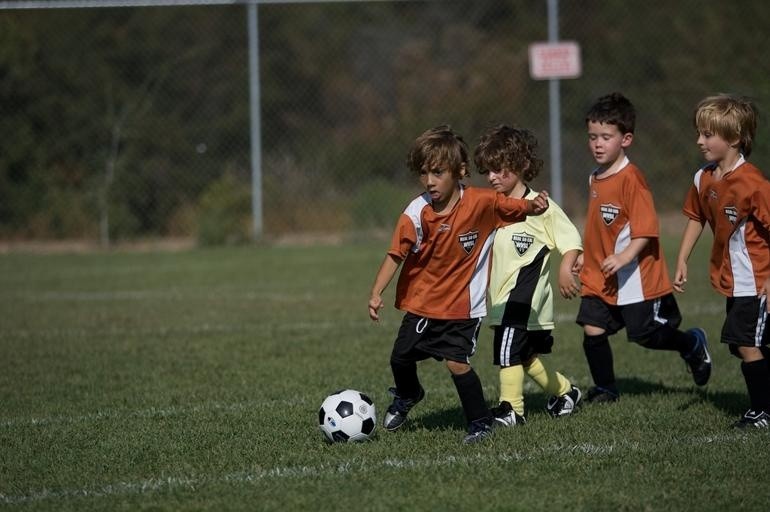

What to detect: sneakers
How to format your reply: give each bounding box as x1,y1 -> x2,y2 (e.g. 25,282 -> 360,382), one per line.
383,385 -> 425,431
494,401 -> 526,427
463,417 -> 496,444
548,385 -> 582,419
583,387 -> 620,404
680,327 -> 711,386
727,409 -> 770,430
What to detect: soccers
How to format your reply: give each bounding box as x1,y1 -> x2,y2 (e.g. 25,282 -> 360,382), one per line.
319,389 -> 377,444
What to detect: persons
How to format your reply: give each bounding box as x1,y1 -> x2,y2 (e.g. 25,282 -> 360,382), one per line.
572,92 -> 711,406
366,124 -> 549,441
471,123 -> 585,424
673,92 -> 770,428
